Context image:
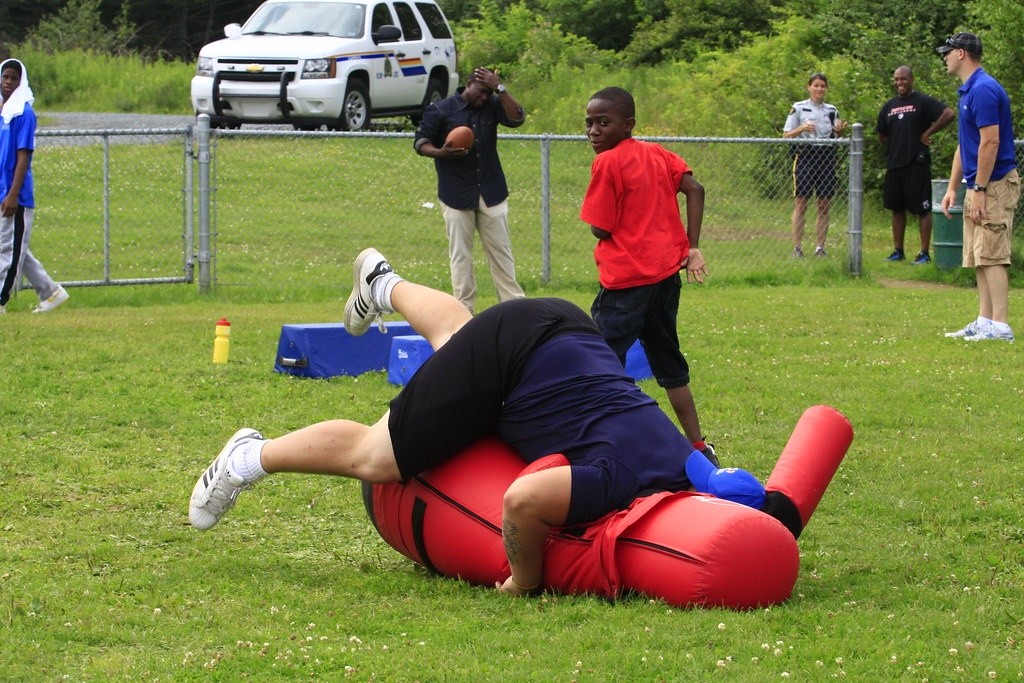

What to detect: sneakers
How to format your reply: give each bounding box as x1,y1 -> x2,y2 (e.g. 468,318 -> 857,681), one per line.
188,427 -> 264,531
791,247 -> 804,258
691,435 -> 720,469
32,284 -> 70,313
884,249 -> 905,261
912,251 -> 931,265
964,320 -> 1015,343
945,320 -> 981,338
342,247 -> 396,337
815,248 -> 826,257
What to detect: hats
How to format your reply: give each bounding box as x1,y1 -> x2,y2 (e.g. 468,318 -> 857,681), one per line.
935,32 -> 982,55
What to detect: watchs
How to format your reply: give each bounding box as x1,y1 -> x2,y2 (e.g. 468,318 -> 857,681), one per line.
494,85 -> 505,94
973,185 -> 985,191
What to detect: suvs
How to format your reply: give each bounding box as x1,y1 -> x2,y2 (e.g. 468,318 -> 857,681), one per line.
191,0 -> 460,132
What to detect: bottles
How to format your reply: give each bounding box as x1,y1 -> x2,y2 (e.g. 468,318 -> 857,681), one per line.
213,317 -> 231,363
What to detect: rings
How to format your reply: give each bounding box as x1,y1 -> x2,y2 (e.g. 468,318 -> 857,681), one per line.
976,213 -> 980,214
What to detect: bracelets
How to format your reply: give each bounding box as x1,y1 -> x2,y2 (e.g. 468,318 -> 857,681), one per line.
512,578 -> 542,592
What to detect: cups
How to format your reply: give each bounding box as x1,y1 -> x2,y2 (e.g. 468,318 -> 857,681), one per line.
807,118 -> 816,131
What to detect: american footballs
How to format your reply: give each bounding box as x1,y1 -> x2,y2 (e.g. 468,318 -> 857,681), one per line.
443,126 -> 475,150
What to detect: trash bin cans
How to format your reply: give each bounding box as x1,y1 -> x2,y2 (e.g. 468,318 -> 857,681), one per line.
931,179 -> 967,266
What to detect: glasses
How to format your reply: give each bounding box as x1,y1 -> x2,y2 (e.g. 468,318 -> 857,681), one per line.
945,37 -> 962,49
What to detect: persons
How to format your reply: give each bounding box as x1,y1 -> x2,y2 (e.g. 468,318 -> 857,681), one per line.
935,32 -> 1019,341
0,58 -> 70,312
413,65 -> 524,313
582,87 -> 720,464
876,66 -> 956,263
189,247 -> 765,595
783,73 -> 847,259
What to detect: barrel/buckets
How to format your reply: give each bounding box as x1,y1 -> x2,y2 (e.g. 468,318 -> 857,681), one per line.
931,179 -> 967,270
